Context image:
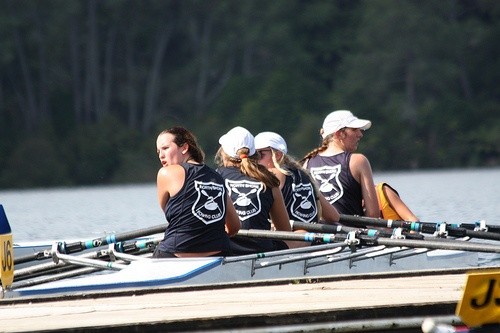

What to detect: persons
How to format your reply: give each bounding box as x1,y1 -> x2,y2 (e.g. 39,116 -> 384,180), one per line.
254,130 -> 341,230
152,126 -> 242,259
213,125 -> 312,249
297,108 -> 380,218
363,180 -> 420,222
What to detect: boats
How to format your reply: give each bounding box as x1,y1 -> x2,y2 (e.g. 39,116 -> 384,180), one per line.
0,217 -> 498,333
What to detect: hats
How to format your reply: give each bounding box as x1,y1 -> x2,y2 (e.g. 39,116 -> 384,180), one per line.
218,126 -> 255,157
252,132 -> 287,155
322,109 -> 371,139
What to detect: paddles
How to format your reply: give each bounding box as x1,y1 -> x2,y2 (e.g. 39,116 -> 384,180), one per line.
0,214 -> 500,291
